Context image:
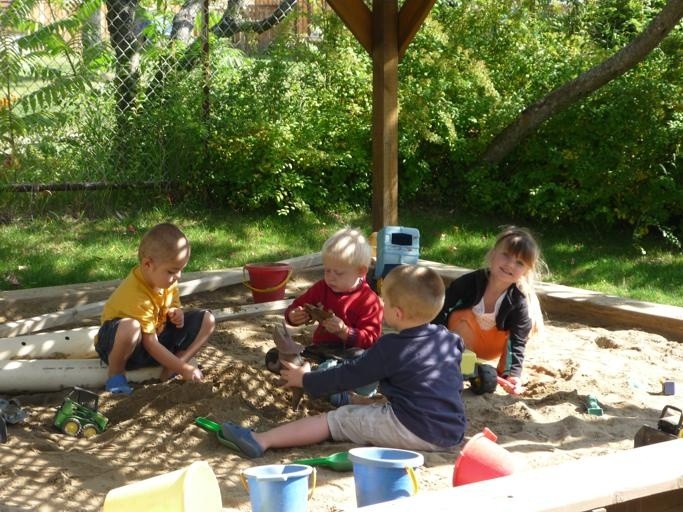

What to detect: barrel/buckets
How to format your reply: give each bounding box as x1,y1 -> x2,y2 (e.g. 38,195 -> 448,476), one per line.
103,459 -> 223,510
348,443 -> 426,507
241,262 -> 297,301
452,427 -> 518,484
242,465 -> 319,512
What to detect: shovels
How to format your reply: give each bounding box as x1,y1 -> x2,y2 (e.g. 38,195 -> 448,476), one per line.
193,416 -> 242,452
293,451 -> 354,472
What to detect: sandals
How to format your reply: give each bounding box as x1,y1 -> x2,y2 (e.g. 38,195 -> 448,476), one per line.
220,421 -> 264,457
317,358 -> 348,406
104,374 -> 131,394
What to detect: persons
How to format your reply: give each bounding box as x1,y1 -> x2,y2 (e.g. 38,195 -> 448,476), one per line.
428,224 -> 545,398
217,263 -> 467,461
265,228 -> 384,406
92,222 -> 215,397
